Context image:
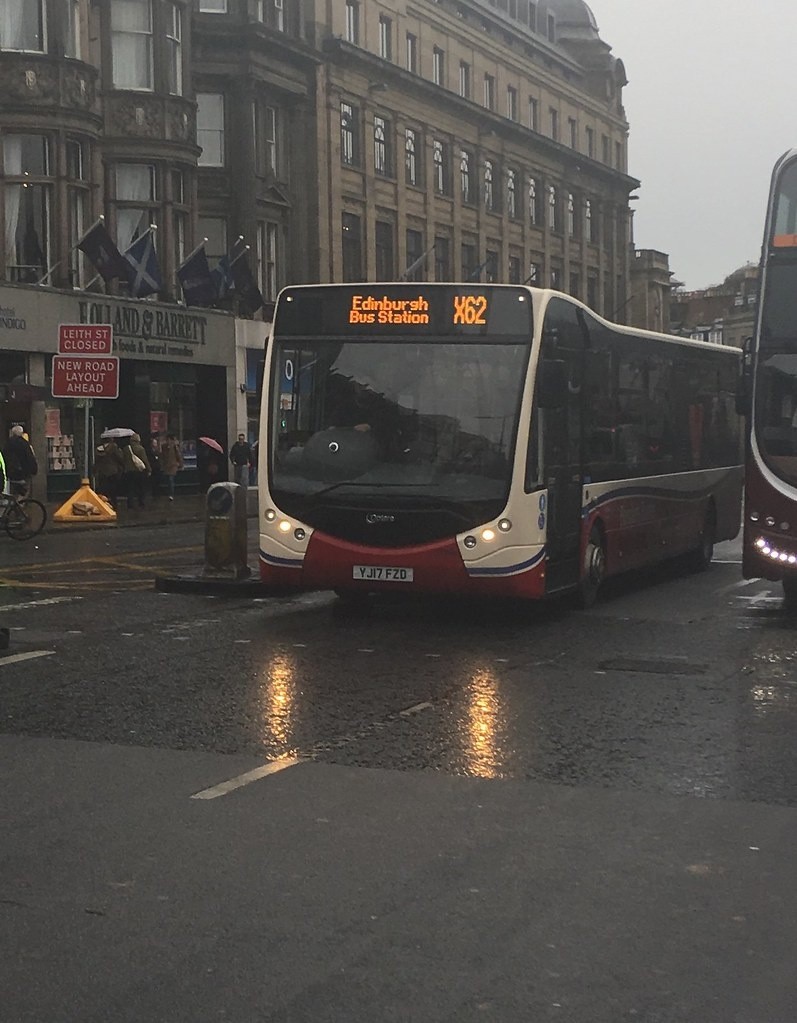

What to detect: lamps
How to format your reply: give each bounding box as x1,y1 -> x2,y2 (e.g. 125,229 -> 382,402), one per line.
564,165 -> 580,176
483,129 -> 495,136
369,83 -> 387,92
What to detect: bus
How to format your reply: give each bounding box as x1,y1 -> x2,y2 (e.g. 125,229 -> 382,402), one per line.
733,143 -> 797,603
258,281 -> 748,603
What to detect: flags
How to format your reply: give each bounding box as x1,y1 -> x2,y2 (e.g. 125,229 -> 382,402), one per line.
211,248 -> 265,320
78,223 -> 125,280
177,245 -> 219,305
122,232 -> 163,298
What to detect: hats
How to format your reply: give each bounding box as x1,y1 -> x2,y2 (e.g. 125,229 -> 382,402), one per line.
130,433 -> 140,441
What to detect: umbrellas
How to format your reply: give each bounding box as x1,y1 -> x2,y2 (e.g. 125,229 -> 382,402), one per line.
199,435 -> 225,454
100,427 -> 137,439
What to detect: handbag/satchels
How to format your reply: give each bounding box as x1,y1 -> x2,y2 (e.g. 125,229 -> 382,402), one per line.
128,445 -> 145,472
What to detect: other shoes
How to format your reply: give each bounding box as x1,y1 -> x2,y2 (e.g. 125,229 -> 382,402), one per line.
169,496 -> 174,501
127,503 -> 136,509
137,502 -> 146,507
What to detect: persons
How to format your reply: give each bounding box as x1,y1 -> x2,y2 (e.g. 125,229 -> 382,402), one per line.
123,434 -> 152,509
2,425 -> 39,510
198,446 -> 224,494
95,437 -> 127,509
229,433 -> 253,484
146,438 -> 161,499
326,366 -> 391,434
159,435 -> 184,502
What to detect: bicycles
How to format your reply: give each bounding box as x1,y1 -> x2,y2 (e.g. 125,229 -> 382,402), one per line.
0,493 -> 47,542
